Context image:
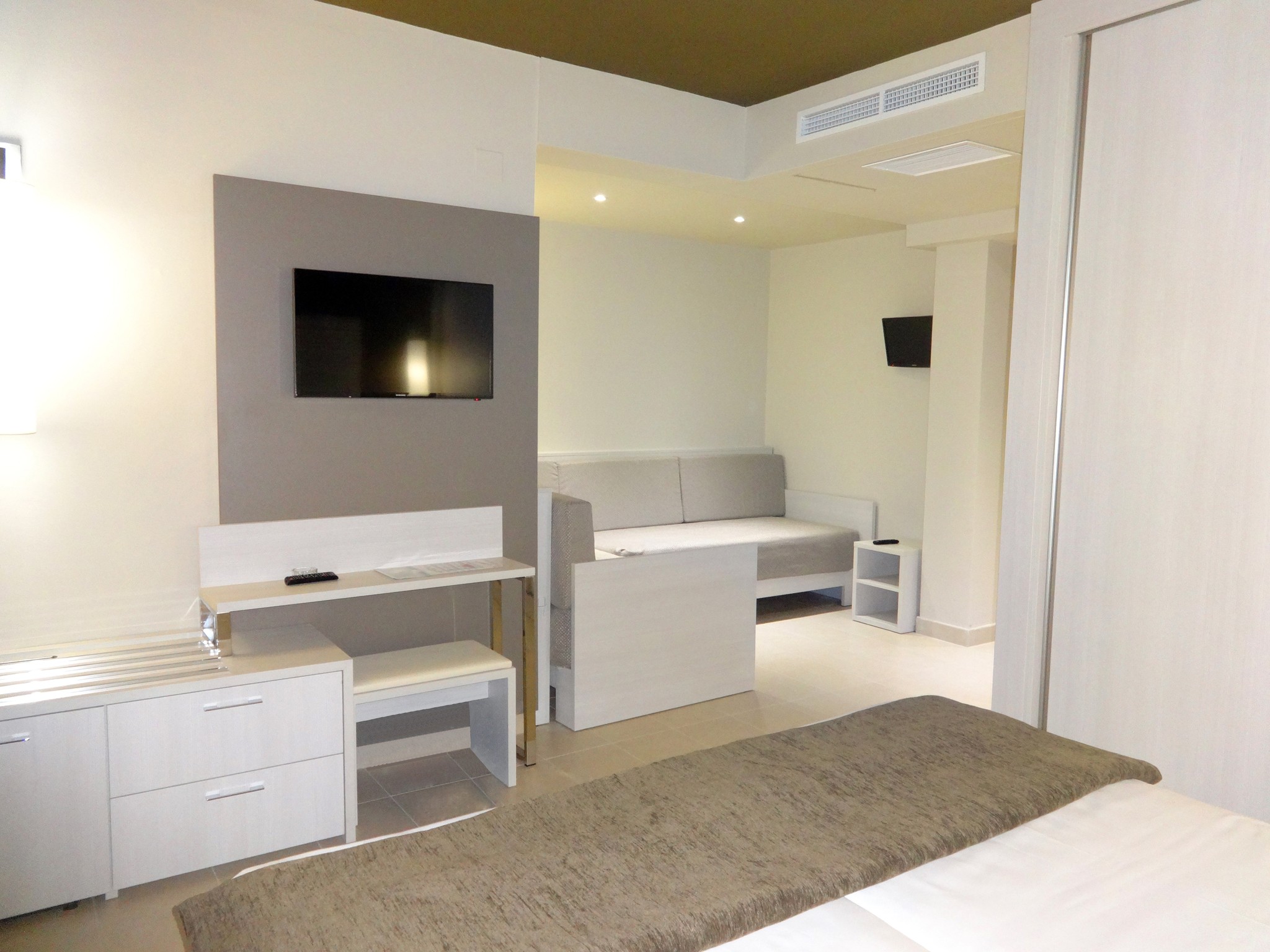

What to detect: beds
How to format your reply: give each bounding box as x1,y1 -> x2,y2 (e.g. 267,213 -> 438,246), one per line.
219,699 -> 1268,952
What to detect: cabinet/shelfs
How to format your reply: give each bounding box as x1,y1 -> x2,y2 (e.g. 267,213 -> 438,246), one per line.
0,622 -> 358,923
201,556 -> 539,790
851,539 -> 922,634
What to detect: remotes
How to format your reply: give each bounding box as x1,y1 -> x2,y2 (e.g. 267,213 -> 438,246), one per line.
284,572 -> 339,586
873,539 -> 900,545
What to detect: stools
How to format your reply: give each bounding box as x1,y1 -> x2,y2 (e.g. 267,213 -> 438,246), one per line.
352,637 -> 518,789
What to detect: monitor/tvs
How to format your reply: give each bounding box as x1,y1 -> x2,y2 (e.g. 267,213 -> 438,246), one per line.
882,315 -> 933,368
292,267 -> 494,401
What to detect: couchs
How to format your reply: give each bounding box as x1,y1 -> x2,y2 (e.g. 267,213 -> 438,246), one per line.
549,448 -> 877,732
